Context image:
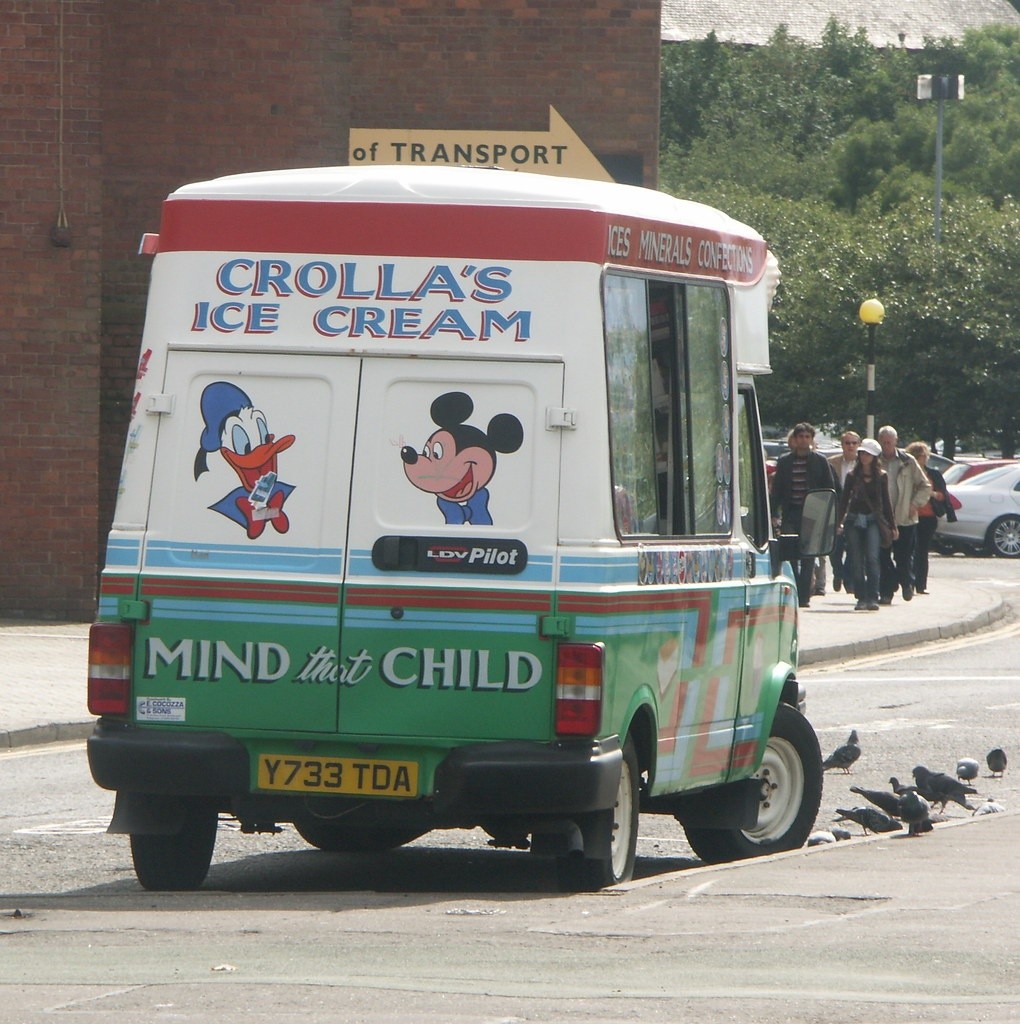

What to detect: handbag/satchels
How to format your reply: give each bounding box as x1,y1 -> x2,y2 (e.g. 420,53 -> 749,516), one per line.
876,513 -> 893,548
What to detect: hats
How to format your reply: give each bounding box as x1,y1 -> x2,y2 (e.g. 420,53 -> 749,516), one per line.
858,437 -> 882,456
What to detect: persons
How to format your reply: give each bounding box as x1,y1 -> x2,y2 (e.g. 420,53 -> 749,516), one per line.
788,429 -> 827,596
906,442 -> 946,594
826,430 -> 860,592
877,426 -> 931,604
839,438 -> 900,610
769,422 -> 835,608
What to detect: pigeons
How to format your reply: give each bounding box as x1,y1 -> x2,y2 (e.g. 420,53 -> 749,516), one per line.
823,729 -> 861,774
955,757 -> 980,785
986,748 -> 1008,777
806,764 -> 1006,848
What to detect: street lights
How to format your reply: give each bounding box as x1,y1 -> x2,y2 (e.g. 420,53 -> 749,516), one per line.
859,298 -> 885,437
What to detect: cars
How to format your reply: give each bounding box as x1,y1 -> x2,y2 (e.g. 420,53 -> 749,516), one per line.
764,437 -> 1020,559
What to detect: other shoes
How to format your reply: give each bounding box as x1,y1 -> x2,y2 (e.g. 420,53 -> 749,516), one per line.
815,586 -> 826,596
916,583 -> 930,595
867,598 -> 880,610
855,598 -> 866,610
833,575 -> 842,591
902,583 -> 913,601
799,601 -> 810,607
880,591 -> 893,604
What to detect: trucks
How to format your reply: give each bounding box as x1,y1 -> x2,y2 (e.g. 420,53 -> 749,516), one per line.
84,164 -> 829,895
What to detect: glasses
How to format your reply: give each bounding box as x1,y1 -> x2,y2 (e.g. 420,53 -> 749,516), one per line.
844,440 -> 859,445
